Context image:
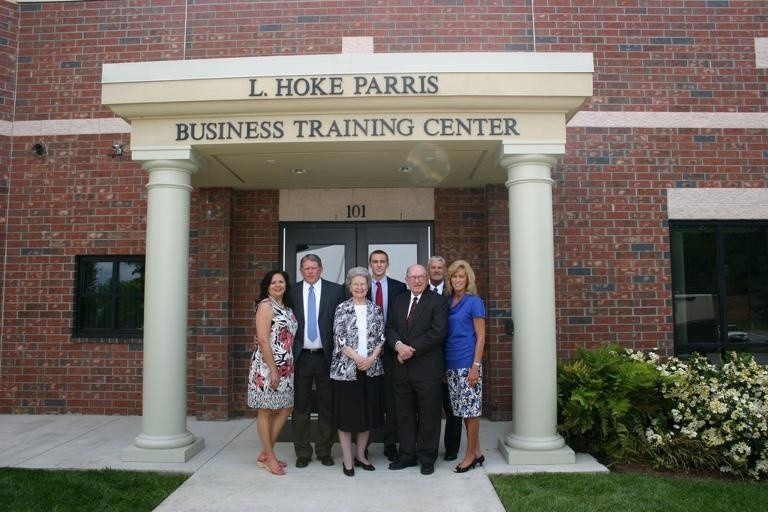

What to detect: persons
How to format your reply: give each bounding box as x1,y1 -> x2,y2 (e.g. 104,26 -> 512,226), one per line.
426,255 -> 462,461
385,264 -> 449,475
253,254 -> 348,468
364,250 -> 408,462
441,259 -> 486,473
329,266 -> 386,477
247,270 -> 298,475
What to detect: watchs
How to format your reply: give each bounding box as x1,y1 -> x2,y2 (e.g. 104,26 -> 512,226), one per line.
373,354 -> 379,360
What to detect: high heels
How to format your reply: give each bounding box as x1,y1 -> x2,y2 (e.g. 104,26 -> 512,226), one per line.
476,455 -> 485,467
453,458 -> 477,473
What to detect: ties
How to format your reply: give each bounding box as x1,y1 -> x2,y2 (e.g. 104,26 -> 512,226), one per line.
376,282 -> 382,307
407,297 -> 418,331
307,285 -> 317,342
433,288 -> 438,293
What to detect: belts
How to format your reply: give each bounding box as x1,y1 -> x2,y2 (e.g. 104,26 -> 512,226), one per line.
302,348 -> 323,354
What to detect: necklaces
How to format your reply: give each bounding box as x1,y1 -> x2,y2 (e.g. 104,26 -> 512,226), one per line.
268,295 -> 285,309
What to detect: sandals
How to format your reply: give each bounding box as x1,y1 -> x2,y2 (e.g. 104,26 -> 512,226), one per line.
257,451 -> 286,468
343,461 -> 354,476
354,457 -> 375,471
264,457 -> 284,475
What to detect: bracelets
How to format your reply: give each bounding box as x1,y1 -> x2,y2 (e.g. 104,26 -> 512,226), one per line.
473,362 -> 480,366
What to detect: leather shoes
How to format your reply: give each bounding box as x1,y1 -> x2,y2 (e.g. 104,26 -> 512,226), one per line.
421,462 -> 434,475
365,448 -> 368,459
318,453 -> 334,465
295,454 -> 311,467
384,446 -> 398,460
389,459 -> 417,470
446,452 -> 457,461
